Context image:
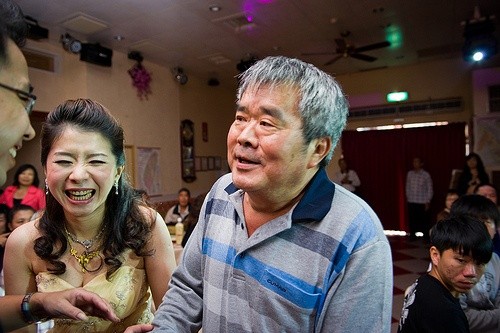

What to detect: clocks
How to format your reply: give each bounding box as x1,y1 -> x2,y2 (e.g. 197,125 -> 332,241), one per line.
180,118 -> 197,183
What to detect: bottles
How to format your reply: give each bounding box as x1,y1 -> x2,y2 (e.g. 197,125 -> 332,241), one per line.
175,217 -> 184,244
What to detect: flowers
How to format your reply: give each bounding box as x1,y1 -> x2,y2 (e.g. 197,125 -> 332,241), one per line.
129,64 -> 153,96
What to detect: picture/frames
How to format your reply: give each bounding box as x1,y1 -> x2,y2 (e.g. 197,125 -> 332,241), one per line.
137,147 -> 163,197
123,145 -> 136,190
195,157 -> 221,171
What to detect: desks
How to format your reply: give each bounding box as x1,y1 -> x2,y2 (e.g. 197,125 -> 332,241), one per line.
169,226 -> 187,267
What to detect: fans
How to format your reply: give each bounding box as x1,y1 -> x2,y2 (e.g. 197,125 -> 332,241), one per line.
303,39 -> 390,67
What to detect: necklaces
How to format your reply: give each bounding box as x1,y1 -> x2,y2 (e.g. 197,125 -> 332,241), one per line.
65,223 -> 105,250
66,235 -> 104,272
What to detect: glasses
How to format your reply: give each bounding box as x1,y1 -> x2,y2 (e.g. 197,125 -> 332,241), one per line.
0,81 -> 37,113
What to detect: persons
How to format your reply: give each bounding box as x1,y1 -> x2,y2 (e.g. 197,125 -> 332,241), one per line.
0,164 -> 46,297
453,153 -> 490,197
164,188 -> 208,249
3,98 -> 178,333
397,209 -> 493,333
0,0 -> 154,333
332,156 -> 360,193
149,56 -> 393,333
427,184 -> 500,333
406,156 -> 432,244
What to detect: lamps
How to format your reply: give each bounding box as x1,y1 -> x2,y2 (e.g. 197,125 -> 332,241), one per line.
173,68 -> 188,85
60,33 -> 82,54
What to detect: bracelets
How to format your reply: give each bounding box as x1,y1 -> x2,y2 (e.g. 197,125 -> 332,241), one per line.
21,291 -> 48,325
